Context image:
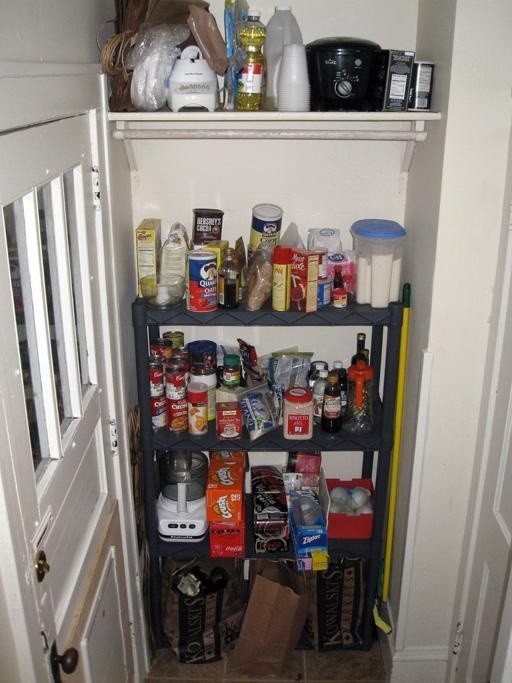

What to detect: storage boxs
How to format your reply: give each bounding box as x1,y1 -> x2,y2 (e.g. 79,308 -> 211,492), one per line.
324,477 -> 376,540
350,219 -> 406,309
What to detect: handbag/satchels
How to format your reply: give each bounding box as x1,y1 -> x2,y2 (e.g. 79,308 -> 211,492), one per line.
161,553 -> 244,664
307,558 -> 374,652
230,561 -> 307,678
97,0 -> 211,111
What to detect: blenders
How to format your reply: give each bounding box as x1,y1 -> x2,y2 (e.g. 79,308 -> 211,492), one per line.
153,451 -> 210,544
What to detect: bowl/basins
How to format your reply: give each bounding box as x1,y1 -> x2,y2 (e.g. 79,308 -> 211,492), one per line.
138,273 -> 186,311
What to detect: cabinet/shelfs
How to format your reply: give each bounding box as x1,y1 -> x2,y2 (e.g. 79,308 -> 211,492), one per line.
132,302 -> 408,649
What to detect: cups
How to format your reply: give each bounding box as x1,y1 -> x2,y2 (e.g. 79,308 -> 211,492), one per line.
276,43 -> 311,112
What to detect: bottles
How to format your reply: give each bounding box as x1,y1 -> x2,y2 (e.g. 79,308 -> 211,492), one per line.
217,248 -> 239,308
308,360 -> 349,432
185,339 -> 217,420
265,2 -> 303,111
236,9 -> 268,112
332,266 -> 351,310
222,353 -> 243,389
346,331 -> 377,435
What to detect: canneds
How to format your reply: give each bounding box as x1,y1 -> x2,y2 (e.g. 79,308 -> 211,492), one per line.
148,329 -> 217,436
